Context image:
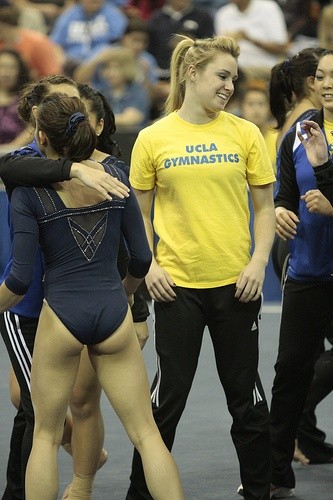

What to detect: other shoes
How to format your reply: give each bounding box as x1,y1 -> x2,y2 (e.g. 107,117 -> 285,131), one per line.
63,441 -> 108,469
62,481 -> 73,500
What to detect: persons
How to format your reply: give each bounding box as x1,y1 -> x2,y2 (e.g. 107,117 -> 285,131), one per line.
0,0 -> 332,499
125,34 -> 277,499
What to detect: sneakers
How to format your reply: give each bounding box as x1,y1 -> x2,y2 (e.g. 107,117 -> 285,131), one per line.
293,439 -> 310,465
237,482 -> 295,497
